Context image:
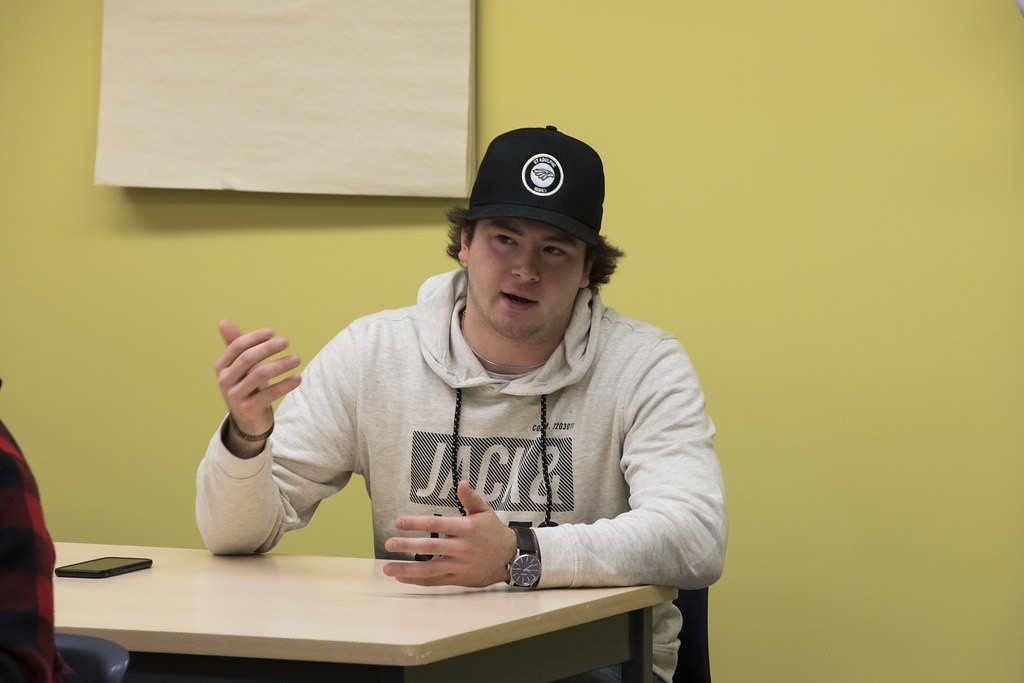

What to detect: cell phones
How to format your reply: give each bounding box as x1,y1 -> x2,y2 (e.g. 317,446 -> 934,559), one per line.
55,558 -> 152,579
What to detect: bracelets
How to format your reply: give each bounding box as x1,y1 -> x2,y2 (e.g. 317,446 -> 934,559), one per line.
195,126 -> 729,682
229,408 -> 274,441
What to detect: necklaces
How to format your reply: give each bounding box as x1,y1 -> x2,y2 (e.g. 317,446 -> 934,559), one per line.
460,308 -> 545,369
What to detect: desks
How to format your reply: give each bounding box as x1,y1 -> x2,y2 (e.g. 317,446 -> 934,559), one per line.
51,540 -> 678,683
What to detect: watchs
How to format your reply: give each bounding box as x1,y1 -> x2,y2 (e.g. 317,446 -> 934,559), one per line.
507,521 -> 541,587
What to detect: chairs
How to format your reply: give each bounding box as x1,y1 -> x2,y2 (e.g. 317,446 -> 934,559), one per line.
672,587 -> 711,683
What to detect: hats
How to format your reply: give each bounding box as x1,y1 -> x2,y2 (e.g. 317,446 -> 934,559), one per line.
466,125 -> 606,243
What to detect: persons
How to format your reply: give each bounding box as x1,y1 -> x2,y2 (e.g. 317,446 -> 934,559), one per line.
0,379 -> 77,683
